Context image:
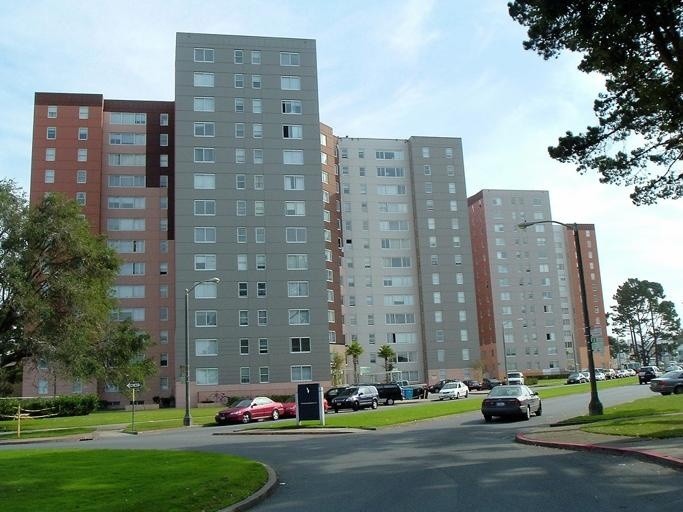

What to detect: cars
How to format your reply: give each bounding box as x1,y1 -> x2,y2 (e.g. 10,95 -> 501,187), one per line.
567,361 -> 683,396
214,395 -> 329,425
396,370 -> 525,400
480,383 -> 543,422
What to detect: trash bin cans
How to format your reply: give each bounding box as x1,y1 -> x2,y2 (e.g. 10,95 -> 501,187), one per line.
295,385 -> 325,426
405,388 -> 414,399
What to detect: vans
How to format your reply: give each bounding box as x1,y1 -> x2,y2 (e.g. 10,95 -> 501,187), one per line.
324,382 -> 405,413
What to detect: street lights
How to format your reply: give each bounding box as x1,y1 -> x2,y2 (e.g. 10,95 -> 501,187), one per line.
183,277 -> 221,427
517,219 -> 604,417
